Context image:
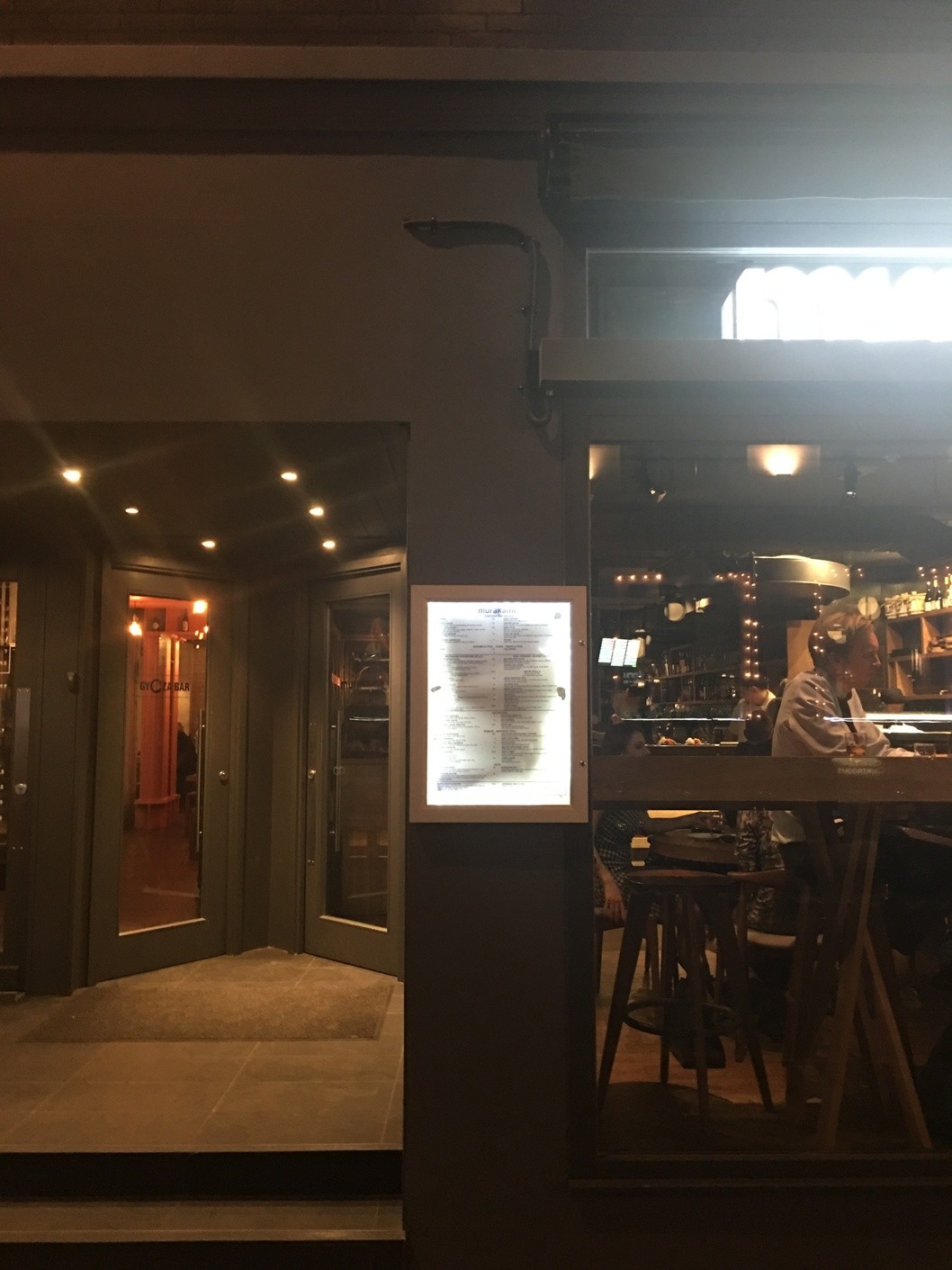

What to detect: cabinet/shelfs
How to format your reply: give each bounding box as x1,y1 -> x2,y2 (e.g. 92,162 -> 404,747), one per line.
128,606 -> 207,640
885,606 -> 952,715
651,640 -> 789,751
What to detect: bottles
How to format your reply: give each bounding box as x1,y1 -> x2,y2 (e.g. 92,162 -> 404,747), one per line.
922,565 -> 952,612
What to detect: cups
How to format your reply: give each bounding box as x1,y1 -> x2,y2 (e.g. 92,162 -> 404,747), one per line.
914,742 -> 936,757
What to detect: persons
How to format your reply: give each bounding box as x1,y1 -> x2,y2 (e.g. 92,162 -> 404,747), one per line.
721,669 -> 783,756
588,720 -> 725,1038
766,601 -> 952,1016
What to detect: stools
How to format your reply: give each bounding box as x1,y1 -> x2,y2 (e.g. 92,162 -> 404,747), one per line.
594,871 -> 783,1159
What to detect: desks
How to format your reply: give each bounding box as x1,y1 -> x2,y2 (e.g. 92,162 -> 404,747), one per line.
585,750 -> 952,1154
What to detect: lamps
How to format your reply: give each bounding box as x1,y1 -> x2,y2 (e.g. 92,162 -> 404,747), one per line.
130,600 -> 140,636
